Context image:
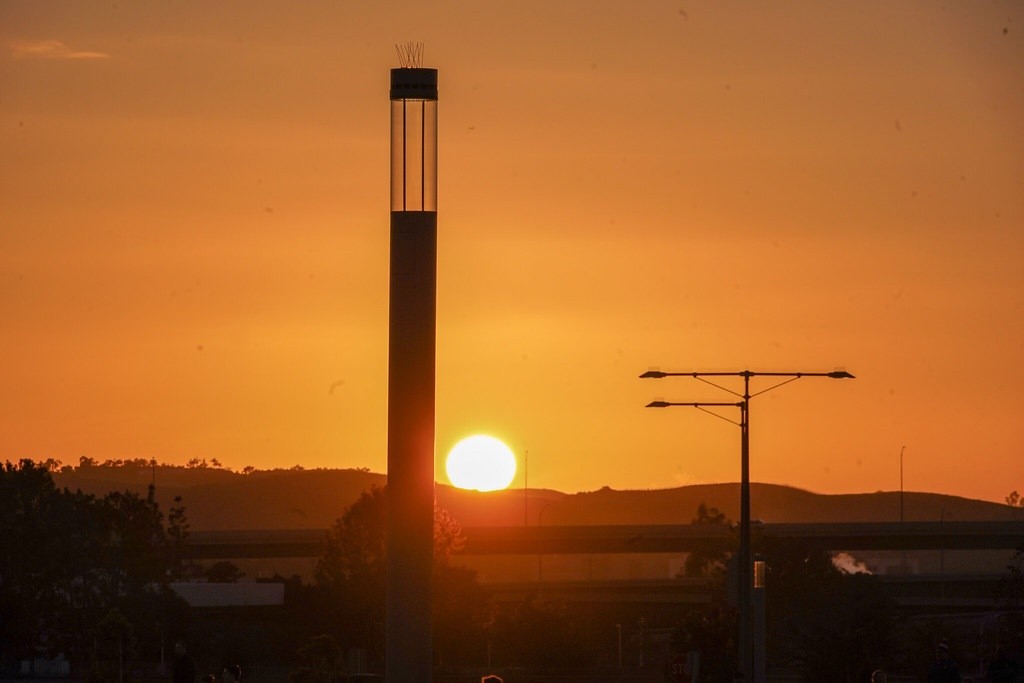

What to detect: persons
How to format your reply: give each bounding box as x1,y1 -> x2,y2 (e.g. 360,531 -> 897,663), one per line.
169,640 -> 246,683
872,641 -> 981,683
